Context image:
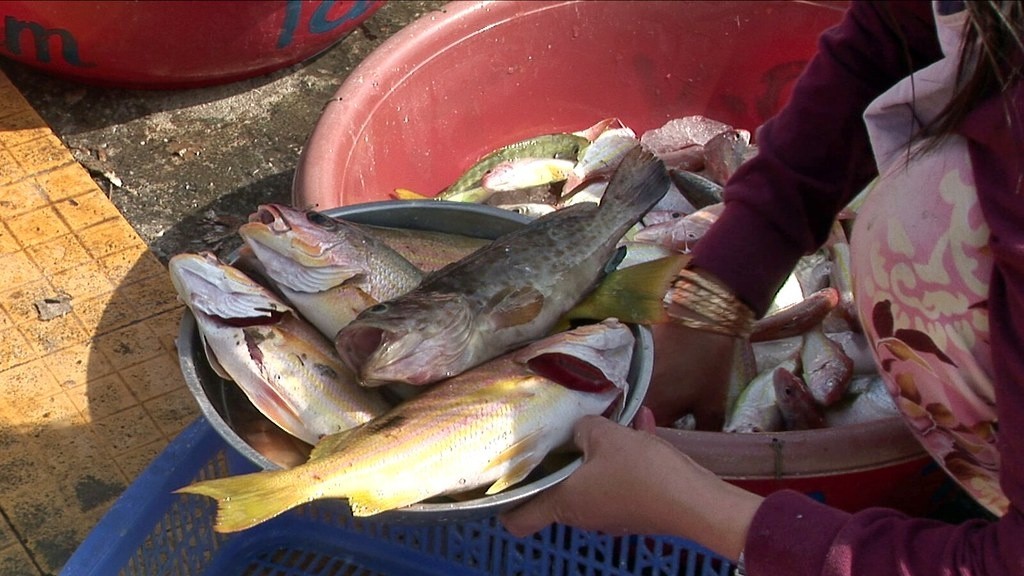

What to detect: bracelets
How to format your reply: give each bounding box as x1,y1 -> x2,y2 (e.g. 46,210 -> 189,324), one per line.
661,268 -> 756,340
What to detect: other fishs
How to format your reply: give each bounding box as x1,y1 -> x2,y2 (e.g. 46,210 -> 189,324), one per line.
166,119 -> 901,532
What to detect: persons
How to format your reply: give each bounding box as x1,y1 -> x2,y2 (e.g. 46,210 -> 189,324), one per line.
498,0 -> 1024,576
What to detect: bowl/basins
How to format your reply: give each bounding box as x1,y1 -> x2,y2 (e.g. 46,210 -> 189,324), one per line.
288,0 -> 933,512
0,0 -> 387,93
178,197 -> 656,526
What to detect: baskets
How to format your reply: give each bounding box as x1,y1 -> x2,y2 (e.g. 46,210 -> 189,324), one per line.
54,412 -> 737,576
